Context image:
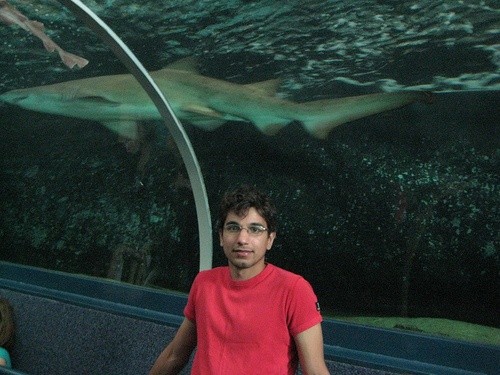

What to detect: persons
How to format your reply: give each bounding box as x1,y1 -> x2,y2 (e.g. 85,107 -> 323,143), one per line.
0,299 -> 13,368
146,191 -> 332,375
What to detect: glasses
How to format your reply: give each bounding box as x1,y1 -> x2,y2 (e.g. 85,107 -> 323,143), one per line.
220,225 -> 271,237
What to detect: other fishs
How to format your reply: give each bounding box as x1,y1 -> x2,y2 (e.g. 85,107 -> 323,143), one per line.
0,0 -> 89,72
